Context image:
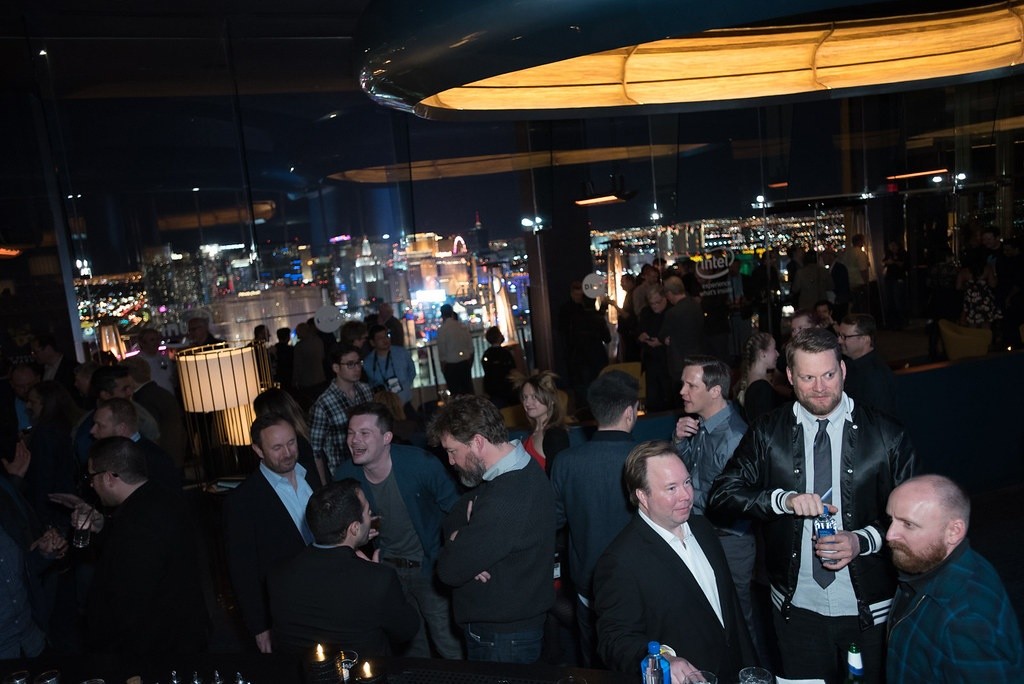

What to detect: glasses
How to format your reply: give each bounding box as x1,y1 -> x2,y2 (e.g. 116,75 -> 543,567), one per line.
83,470 -> 119,483
338,360 -> 364,369
837,332 -> 868,340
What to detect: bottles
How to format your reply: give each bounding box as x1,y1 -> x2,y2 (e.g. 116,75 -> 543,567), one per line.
843,639 -> 870,684
641,641 -> 671,684
813,506 -> 841,565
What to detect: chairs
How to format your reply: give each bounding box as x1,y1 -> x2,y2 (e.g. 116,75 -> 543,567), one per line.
938,319 -> 993,360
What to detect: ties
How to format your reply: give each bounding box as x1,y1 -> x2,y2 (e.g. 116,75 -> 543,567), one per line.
813,418 -> 836,588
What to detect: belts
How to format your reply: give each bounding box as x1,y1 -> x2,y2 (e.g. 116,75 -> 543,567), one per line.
385,558 -> 420,568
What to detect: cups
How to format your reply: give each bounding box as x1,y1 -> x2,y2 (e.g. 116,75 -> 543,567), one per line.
334,651 -> 358,684
685,670 -> 719,684
72,505 -> 95,548
738,666 -> 773,684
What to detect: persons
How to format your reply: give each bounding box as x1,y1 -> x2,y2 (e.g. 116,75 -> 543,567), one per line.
333,402 -> 464,660
479,328 -> 523,406
266,477 -> 421,657
704,324 -> 926,684
519,373 -> 569,558
556,246 -> 785,415
0,323 -> 215,662
883,473 -> 1024,684
785,221 -> 1024,351
669,354 -> 762,669
729,297 -> 902,419
187,317 -> 228,352
437,306 -> 477,395
420,394 -> 558,665
310,347 -> 377,486
221,412 -> 322,654
252,388 -> 315,464
243,302 -> 422,420
548,369 -> 645,670
587,439 -> 744,684
